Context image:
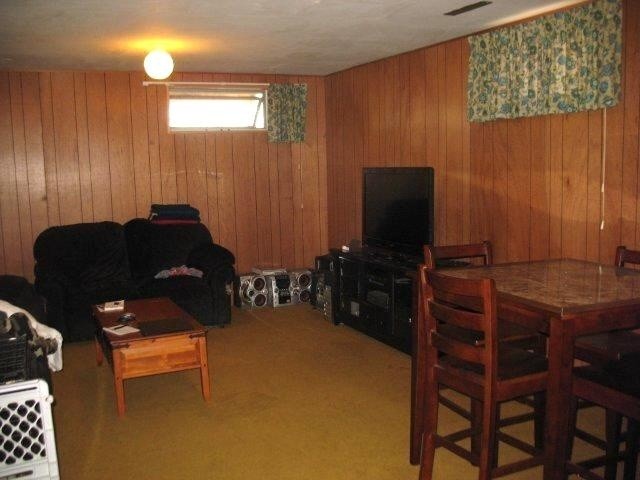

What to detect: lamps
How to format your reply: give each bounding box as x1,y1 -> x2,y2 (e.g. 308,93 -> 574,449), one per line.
143,48 -> 175,80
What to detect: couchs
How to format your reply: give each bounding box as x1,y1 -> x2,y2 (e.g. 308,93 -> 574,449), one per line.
34,218 -> 236,343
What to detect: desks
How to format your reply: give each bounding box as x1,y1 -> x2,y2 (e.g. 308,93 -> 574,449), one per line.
91,296 -> 212,416
424,258 -> 640,478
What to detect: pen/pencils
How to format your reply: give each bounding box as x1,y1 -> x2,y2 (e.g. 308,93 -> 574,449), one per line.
114,325 -> 127,330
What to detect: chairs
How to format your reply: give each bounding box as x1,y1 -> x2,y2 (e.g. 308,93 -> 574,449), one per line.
414,262 -> 550,478
423,242 -> 548,467
570,361 -> 640,479
573,244 -> 640,452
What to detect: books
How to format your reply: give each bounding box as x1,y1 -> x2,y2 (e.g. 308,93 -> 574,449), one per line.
103,325 -> 140,336
104,299 -> 124,312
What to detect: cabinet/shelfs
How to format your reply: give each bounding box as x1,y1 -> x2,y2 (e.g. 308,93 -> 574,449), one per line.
330,258 -> 470,357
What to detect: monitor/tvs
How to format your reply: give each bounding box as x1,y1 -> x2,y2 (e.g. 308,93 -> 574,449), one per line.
361,166 -> 434,263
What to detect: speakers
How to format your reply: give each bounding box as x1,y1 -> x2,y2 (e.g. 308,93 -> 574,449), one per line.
233,267 -> 313,308
